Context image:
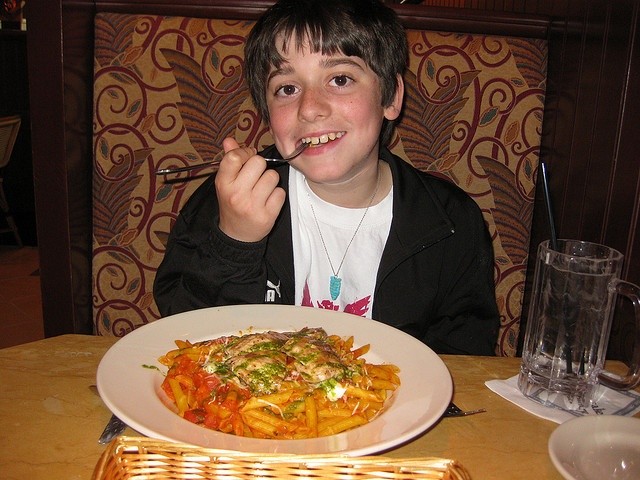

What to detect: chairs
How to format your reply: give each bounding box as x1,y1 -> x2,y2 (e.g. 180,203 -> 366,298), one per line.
93,2 -> 548,359
1,113 -> 23,251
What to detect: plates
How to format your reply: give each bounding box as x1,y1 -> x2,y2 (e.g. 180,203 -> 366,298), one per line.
96,304 -> 454,457
547,415 -> 640,480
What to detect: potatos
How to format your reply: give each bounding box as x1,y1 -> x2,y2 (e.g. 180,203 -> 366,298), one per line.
161,333 -> 400,440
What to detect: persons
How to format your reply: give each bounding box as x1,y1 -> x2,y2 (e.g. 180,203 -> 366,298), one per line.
153,0 -> 499,356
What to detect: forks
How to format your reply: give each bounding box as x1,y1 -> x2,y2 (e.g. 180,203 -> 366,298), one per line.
444,402 -> 487,416
154,142 -> 310,178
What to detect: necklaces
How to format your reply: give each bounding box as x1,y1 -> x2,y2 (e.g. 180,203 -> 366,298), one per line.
301,157 -> 381,299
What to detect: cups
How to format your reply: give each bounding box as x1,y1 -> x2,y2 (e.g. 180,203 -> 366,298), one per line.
517,238 -> 640,413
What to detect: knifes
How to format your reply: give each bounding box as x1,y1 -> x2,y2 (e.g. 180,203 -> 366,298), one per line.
98,413 -> 128,445
